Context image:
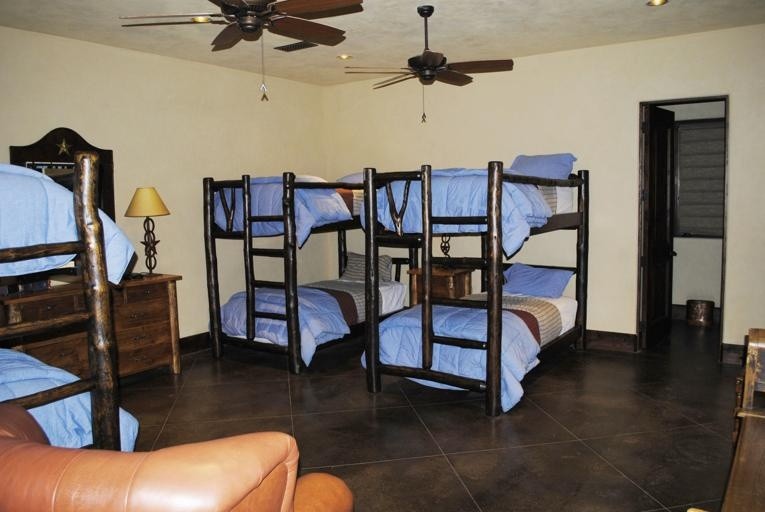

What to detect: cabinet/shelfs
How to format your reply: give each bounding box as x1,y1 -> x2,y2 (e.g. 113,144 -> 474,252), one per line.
0,272 -> 183,387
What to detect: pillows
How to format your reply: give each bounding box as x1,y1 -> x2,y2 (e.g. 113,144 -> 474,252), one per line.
509,154 -> 579,180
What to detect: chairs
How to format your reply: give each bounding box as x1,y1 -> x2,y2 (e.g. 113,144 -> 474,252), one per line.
0,401 -> 356,511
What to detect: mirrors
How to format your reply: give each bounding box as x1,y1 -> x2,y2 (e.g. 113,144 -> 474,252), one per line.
9,124 -> 115,225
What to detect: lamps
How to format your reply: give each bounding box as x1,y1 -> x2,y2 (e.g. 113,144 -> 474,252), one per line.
124,187 -> 172,279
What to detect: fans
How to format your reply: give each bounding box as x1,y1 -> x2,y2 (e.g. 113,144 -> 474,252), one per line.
118,0 -> 363,102
344,4 -> 515,122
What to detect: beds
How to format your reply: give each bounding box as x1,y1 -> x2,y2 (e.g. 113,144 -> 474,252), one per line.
1,148 -> 142,456
